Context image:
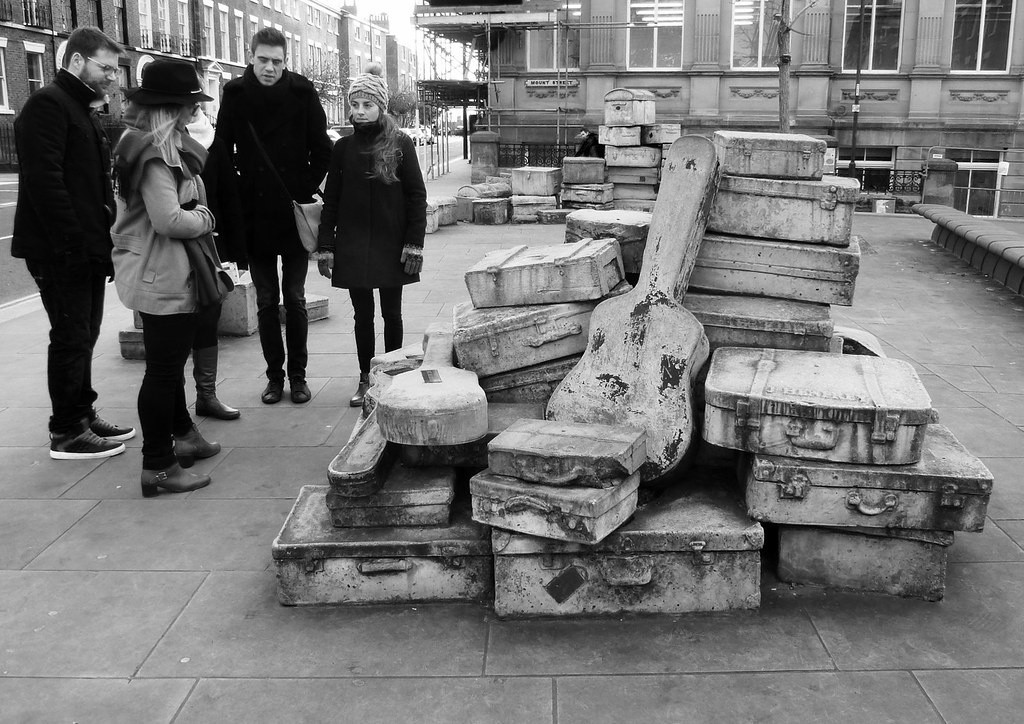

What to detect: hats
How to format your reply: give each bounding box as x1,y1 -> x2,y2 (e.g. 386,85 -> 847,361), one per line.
124,59 -> 214,106
347,62 -> 389,114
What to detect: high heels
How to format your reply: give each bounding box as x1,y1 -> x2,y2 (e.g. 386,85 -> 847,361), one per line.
173,422 -> 220,468
140,462 -> 211,497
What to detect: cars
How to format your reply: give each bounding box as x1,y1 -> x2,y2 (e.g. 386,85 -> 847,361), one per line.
438,127 -> 452,135
454,126 -> 465,136
326,123 -> 438,147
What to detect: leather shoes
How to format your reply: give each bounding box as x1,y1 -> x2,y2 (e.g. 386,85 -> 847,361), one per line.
290,380 -> 311,403
262,380 -> 283,403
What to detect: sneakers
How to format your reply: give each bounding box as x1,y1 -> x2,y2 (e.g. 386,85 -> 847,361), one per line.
49,419 -> 125,460
89,414 -> 135,441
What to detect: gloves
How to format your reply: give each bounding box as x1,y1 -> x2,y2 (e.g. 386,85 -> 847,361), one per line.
401,243 -> 423,275
318,250 -> 334,279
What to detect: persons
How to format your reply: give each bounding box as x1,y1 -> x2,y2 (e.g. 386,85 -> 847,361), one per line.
10,28 -> 135,460
207,27 -> 332,404
312,61 -> 428,406
109,59 -> 240,498
575,128 -> 605,158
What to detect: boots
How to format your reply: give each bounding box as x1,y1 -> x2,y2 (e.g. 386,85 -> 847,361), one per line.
349,373 -> 369,406
192,343 -> 241,420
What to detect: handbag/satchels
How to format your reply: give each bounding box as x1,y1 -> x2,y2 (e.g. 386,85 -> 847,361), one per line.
183,230 -> 235,307
291,193 -> 323,254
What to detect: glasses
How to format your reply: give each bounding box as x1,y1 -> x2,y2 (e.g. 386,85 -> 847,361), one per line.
87,56 -> 122,77
185,104 -> 200,114
